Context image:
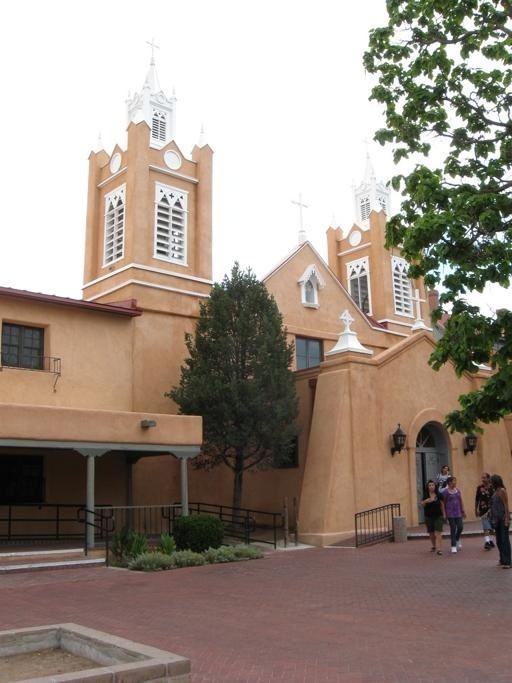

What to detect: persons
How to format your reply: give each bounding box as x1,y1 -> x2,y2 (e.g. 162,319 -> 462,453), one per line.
441,476 -> 466,554
474,473 -> 496,550
480,474 -> 511,569
436,465 -> 452,522
420,479 -> 445,555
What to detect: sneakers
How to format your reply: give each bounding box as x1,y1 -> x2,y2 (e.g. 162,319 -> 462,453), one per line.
430,547 -> 435,552
457,542 -> 462,548
485,541 -> 494,549
495,559 -> 512,568
437,550 -> 443,555
451,547 -> 457,553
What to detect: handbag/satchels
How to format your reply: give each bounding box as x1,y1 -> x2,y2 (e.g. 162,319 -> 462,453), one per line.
490,517 -> 498,528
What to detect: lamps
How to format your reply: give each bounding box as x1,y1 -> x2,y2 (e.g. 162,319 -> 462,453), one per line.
464,432 -> 478,456
391,422 -> 407,456
